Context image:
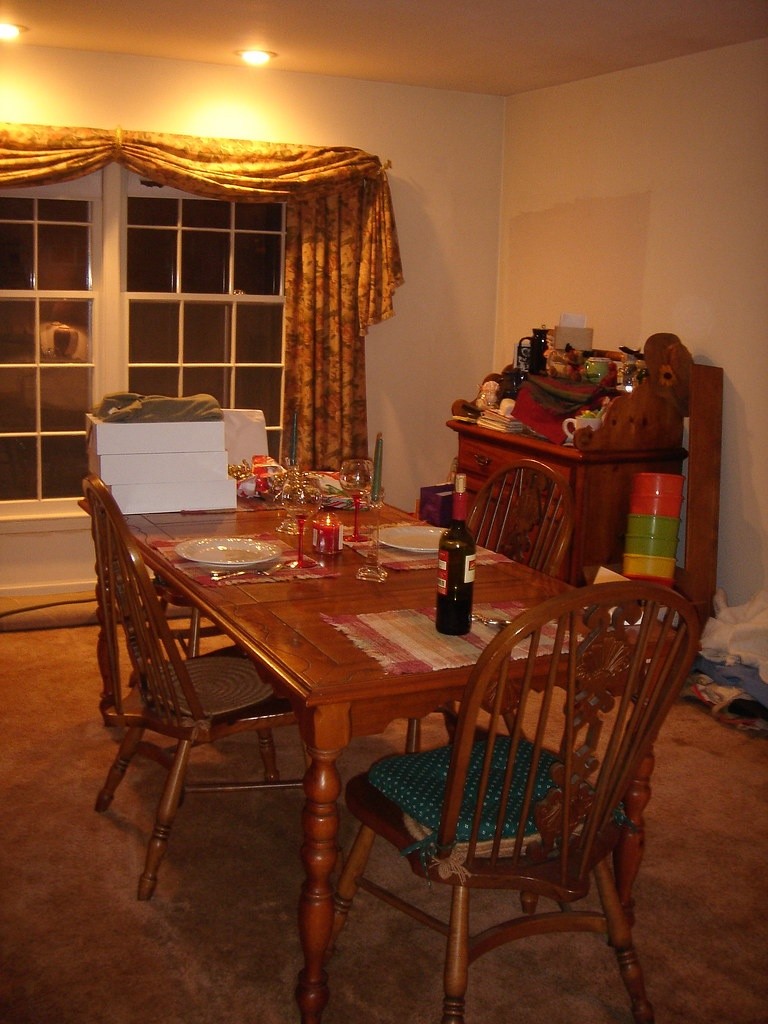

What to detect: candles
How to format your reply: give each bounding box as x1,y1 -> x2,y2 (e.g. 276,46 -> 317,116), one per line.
313,512 -> 342,554
372,432 -> 383,500
290,409 -> 297,465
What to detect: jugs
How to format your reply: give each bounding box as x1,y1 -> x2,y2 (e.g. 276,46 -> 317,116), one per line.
519,324 -> 552,376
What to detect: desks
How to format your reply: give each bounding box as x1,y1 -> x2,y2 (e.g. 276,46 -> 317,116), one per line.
122,497 -> 697,1024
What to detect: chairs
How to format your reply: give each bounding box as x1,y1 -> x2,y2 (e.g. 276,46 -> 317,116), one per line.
323,581 -> 702,1024
464,458 -> 574,578
130,408 -> 269,687
80,474 -> 312,902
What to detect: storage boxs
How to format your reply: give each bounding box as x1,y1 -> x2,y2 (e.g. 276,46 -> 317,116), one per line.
84,413 -> 238,514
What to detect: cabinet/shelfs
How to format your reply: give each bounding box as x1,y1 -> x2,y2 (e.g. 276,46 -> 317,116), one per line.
447,349 -> 724,640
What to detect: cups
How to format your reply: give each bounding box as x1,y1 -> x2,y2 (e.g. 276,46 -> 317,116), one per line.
585,356 -> 611,385
622,471 -> 686,590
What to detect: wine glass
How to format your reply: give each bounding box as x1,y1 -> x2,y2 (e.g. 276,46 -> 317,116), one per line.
282,472 -> 318,568
272,474 -> 309,535
339,459 -> 373,543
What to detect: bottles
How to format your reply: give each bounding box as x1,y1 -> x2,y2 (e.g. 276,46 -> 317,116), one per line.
436,473 -> 476,635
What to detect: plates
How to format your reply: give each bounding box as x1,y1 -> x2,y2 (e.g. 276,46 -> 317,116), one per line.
175,536 -> 282,567
374,524 -> 455,553
